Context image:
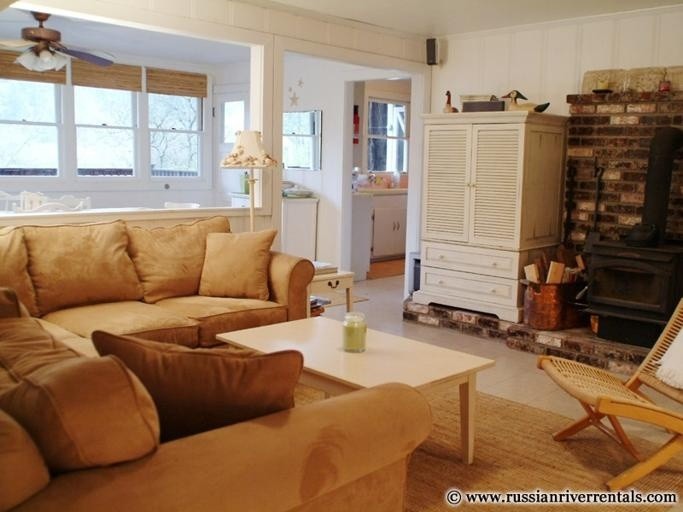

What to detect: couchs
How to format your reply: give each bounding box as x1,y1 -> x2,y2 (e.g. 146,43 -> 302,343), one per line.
0,251 -> 433,512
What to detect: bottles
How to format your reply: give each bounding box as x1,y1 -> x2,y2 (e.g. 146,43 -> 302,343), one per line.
240,170 -> 249,194
342,312 -> 367,354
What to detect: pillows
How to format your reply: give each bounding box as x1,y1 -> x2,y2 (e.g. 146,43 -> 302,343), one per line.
20,219 -> 144,317
198,227 -> 276,300
0,225 -> 41,317
90,329 -> 304,431
0,314 -> 162,471
124,216 -> 232,303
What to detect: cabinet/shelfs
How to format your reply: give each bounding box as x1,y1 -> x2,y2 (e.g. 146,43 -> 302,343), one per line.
226,191 -> 319,261
412,112 -> 568,325
350,194 -> 408,282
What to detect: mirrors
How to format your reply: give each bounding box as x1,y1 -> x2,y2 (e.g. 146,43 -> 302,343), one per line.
281,109 -> 321,173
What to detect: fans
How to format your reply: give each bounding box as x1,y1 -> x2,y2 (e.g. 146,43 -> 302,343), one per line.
0,11 -> 116,66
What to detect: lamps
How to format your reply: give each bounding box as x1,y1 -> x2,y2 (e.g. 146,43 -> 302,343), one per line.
12,39 -> 65,72
219,130 -> 277,234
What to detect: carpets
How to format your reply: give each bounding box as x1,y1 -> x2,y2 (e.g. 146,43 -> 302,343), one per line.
308,291 -> 368,307
282,384 -> 683,512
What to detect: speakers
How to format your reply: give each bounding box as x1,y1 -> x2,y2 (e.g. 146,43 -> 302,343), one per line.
427,38 -> 438,65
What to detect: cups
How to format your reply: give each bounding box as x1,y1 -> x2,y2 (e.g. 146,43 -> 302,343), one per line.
399,171 -> 407,188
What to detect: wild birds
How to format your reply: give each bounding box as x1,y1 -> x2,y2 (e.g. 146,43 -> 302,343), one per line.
442,90 -> 459,113
500,89 -> 550,114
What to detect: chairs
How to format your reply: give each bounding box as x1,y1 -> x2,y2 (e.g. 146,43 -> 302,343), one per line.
537,298 -> 683,494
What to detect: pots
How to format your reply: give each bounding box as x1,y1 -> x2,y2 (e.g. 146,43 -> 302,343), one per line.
519,278 -> 583,331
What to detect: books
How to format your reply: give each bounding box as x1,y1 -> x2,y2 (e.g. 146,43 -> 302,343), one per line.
457,95 -> 499,102
311,260 -> 338,275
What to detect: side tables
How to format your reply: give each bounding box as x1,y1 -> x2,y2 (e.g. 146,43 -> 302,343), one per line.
306,270 -> 355,318
